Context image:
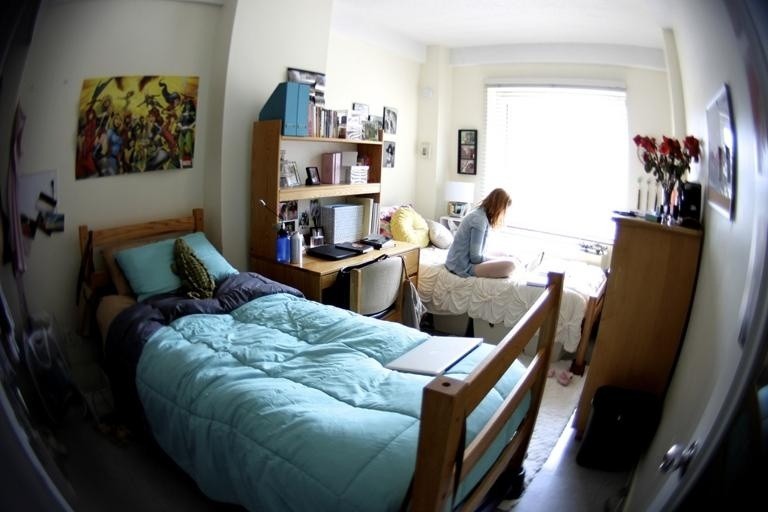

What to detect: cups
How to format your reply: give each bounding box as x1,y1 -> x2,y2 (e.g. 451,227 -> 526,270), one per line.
310,236 -> 324,246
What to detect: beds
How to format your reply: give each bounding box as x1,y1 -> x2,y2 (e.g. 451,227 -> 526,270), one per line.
379,207 -> 608,375
77,205 -> 566,511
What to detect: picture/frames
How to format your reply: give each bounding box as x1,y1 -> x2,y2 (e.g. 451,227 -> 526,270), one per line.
304,165 -> 320,186
703,82 -> 735,222
457,128 -> 476,175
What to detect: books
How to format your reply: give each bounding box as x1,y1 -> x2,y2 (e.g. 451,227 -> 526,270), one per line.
308,104 -> 339,139
322,150 -> 369,185
321,197 -> 379,245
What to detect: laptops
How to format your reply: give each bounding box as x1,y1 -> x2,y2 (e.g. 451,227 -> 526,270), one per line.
510,251 -> 545,277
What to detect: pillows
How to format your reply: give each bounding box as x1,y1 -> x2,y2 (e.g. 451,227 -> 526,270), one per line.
379,204 -> 453,251
114,228 -> 237,299
95,233 -> 187,294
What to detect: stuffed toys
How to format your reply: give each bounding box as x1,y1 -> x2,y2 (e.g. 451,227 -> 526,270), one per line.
171,239 -> 215,299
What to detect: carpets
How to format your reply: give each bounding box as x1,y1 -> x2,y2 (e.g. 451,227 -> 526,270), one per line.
501,357 -> 588,509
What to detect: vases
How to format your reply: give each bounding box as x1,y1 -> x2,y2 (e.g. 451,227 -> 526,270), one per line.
652,177 -> 676,223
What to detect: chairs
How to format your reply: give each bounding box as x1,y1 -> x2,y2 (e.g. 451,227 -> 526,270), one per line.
337,254 -> 404,322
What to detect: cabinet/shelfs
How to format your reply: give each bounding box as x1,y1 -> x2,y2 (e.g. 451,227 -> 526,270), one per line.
253,120 -> 385,259
572,215 -> 699,441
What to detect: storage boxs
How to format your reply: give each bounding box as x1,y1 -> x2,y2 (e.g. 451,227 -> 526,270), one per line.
524,332 -> 565,362
471,316 -> 511,345
431,308 -> 469,336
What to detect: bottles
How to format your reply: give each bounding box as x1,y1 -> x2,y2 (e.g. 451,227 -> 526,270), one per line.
635,177 -> 659,214
276,229 -> 290,262
290,231 -> 303,265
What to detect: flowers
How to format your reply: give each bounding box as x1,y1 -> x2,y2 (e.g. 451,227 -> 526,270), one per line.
631,128 -> 702,181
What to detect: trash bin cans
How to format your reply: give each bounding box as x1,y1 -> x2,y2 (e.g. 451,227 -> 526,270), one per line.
575,384 -> 662,473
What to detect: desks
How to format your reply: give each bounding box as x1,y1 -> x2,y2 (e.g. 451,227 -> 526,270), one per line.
257,241 -> 422,322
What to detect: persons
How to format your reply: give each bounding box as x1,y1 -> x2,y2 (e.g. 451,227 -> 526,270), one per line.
386,145 -> 392,168
78,83 -> 193,176
445,188 -> 518,279
